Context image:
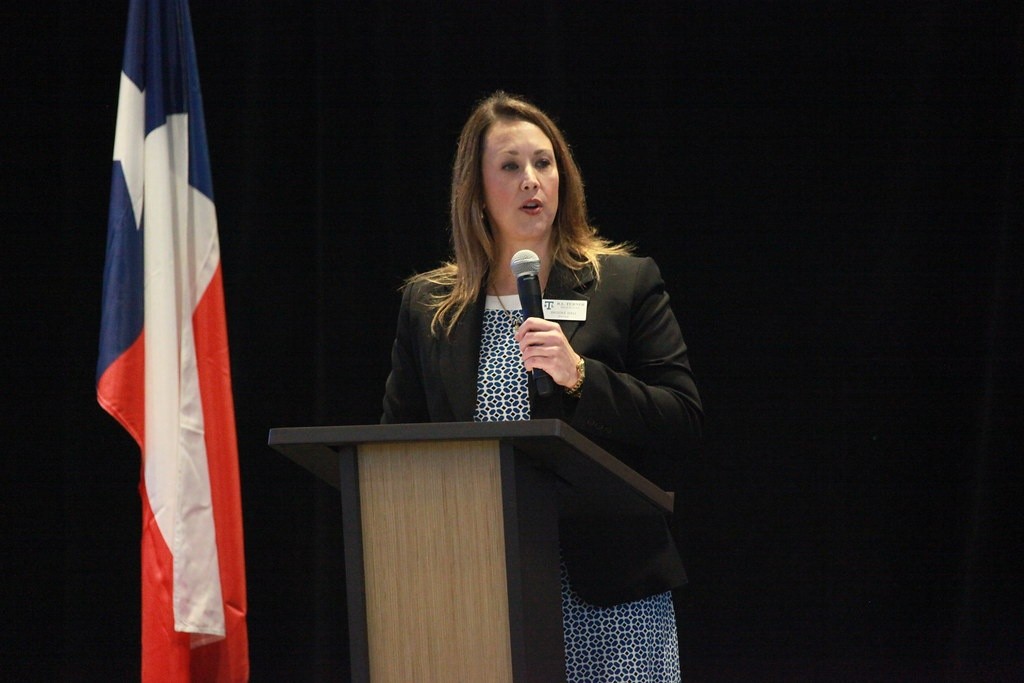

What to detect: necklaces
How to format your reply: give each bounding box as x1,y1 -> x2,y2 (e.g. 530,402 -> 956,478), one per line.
488,255 -> 546,335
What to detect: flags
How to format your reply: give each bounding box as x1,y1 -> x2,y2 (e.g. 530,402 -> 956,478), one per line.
101,0 -> 250,682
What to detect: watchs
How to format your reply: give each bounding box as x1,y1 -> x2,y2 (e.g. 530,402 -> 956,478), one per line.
566,354 -> 584,397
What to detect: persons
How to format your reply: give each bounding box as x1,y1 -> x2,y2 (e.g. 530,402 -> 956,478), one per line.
381,91 -> 705,681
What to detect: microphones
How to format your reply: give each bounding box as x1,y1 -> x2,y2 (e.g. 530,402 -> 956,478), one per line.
509,249 -> 554,397
